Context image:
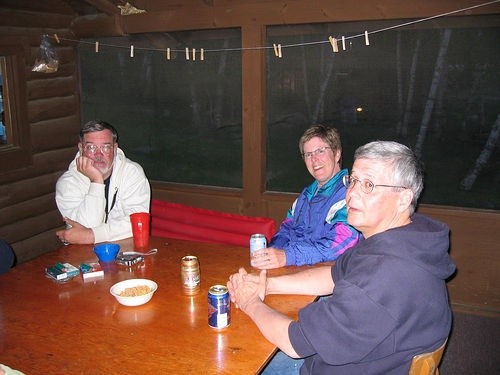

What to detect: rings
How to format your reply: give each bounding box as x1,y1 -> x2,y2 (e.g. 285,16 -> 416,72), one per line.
63,240 -> 65,243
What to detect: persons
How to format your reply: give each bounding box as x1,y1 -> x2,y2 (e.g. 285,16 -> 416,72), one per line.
253,124 -> 365,269
227,141 -> 457,375
55,119 -> 150,244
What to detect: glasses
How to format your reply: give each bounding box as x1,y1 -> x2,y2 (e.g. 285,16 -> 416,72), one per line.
342,174 -> 405,193
301,147 -> 331,160
81,143 -> 114,155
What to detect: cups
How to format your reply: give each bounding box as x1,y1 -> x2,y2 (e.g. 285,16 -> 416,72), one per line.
130,213 -> 150,248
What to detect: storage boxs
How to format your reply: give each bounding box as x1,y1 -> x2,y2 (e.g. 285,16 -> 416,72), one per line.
45,263 -> 80,280
81,262 -> 104,279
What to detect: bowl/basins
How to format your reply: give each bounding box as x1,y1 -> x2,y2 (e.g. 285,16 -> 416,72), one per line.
93,243 -> 120,262
110,278 -> 158,306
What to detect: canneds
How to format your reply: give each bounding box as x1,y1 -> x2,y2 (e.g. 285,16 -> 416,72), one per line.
180,255 -> 200,293
208,285 -> 231,329
250,233 -> 266,266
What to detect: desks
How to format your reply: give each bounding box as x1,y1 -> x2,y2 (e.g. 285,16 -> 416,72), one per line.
0,234 -> 318,374
